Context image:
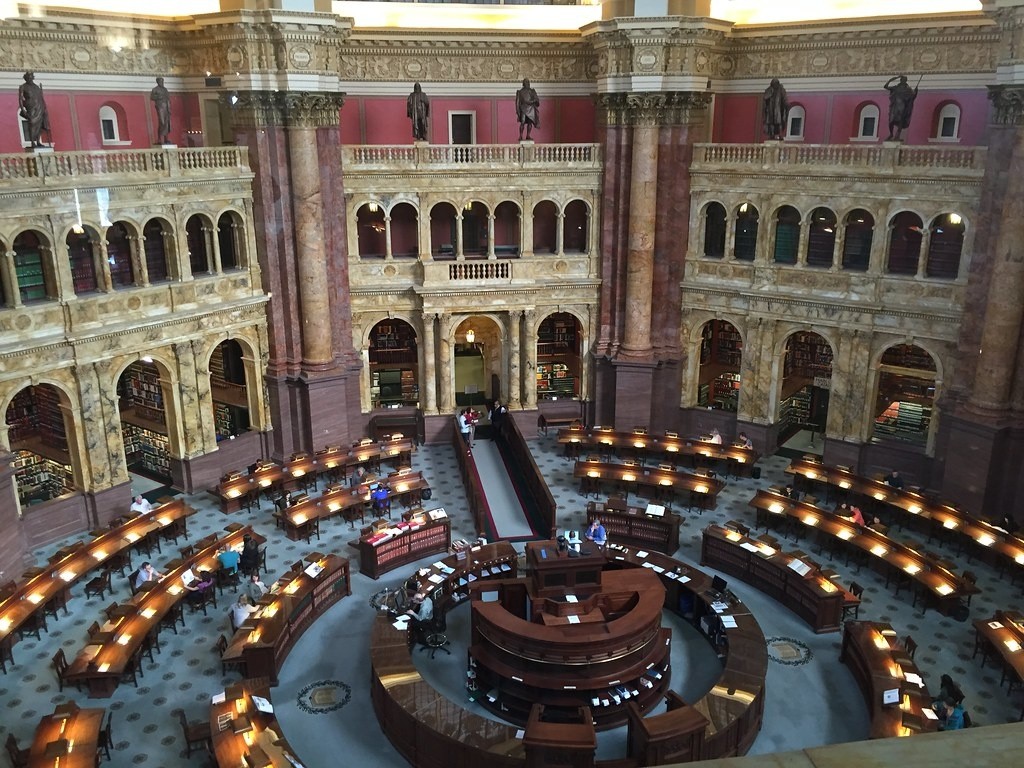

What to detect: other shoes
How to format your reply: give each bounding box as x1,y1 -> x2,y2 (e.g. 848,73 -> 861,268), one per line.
473,444 -> 475,446
471,447 -> 473,448
490,438 -> 495,442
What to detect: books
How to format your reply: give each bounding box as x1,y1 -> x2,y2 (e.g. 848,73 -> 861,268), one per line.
366,521 -> 468,566
273,566 -> 344,662
590,514 -> 669,542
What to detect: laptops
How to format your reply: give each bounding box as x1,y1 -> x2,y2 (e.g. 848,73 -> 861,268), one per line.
395,589 -> 413,612
565,530 -> 579,540
704,575 -> 727,601
181,569 -> 201,587
370,484 -> 378,493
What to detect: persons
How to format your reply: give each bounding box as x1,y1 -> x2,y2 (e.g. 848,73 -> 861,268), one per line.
350,467 -> 389,509
704,427 -> 752,450
567,520 -> 607,552
1000,514 -> 1020,534
406,592 -> 433,644
478,530 -> 487,546
184,534 -> 271,628
18,72 -> 48,148
135,562 -> 166,589
407,83 -> 430,142
515,78 -> 541,140
251,459 -> 273,500
780,471 -> 903,535
936,698 -> 964,731
130,493 -> 153,515
278,491 -> 299,510
150,77 -> 172,144
460,401 -> 506,449
762,77 -> 788,141
884,75 -> 918,141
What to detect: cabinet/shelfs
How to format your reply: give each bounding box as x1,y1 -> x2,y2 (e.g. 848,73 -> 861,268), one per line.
700,319 -> 742,412
3,339 -> 237,500
872,343 -> 937,444
538,312 -> 581,397
367,318 -> 420,408
777,329 -> 835,440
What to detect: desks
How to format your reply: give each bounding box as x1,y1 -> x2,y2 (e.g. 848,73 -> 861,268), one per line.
0,426 -> 1024,768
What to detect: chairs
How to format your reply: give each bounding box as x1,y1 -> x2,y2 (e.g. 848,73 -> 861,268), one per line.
756,507 -> 776,534
298,514 -> 321,545
403,486 -> 423,510
216,634 -> 241,676
133,527 -> 162,559
840,581 -> 863,621
118,643 -> 144,688
341,501 -> 365,528
298,469 -> 318,495
0,632 -> 15,675
784,513 -> 807,543
43,585 -> 67,621
263,476 -> 285,501
17,602 -> 48,642
96,711 -> 113,761
904,634 -> 918,660
885,562 -> 911,594
584,475 -> 600,498
963,711 -> 973,729
364,454 -> 381,475
624,479 -> 639,498
372,497 -> 392,520
999,656 -> 1022,697
418,607 -> 450,658
972,629 -> 1003,669
162,514 -> 188,544
394,449 -> 411,469
329,463 -> 347,484
172,706 -> 213,759
911,581 -> 935,615
139,624 -> 161,664
239,486 -> 261,513
52,648 -> 83,694
961,570 -> 977,607
4,732 -> 31,768
108,548 -> 134,577
85,569 -> 113,602
160,597 -> 186,635
188,581 -> 217,616
689,489 -> 707,515
